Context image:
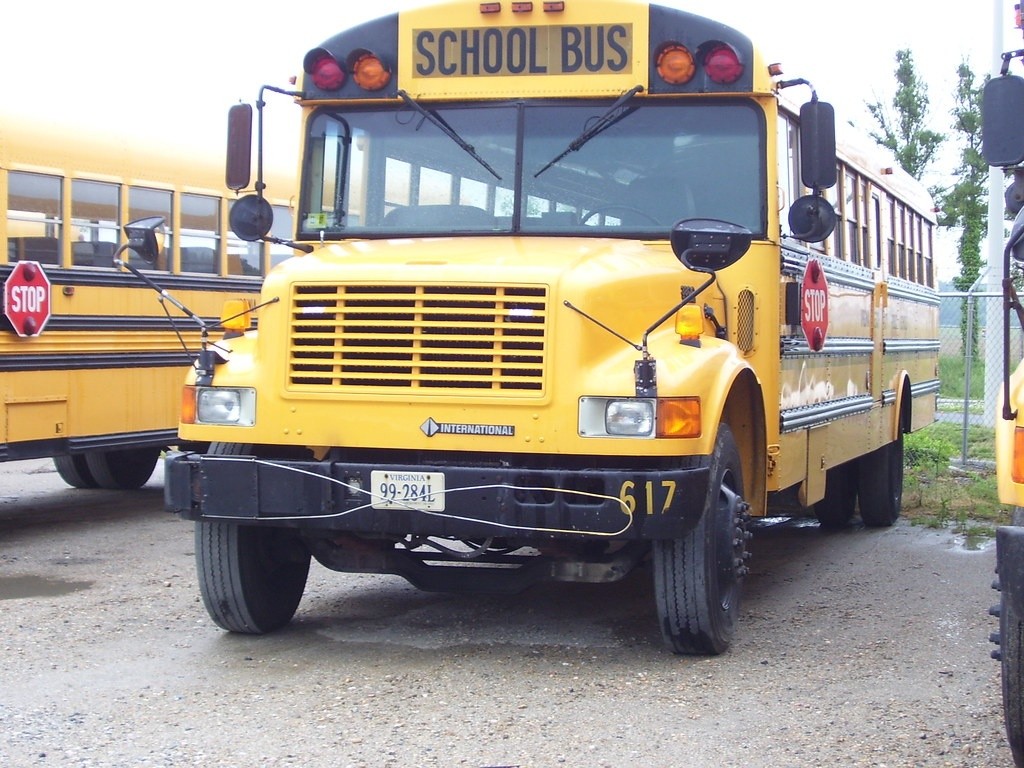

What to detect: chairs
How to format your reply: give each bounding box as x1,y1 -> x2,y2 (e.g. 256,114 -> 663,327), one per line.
8,235 -> 217,275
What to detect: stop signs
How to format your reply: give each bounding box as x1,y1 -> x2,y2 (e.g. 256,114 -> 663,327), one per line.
4,258 -> 52,338
800,259 -> 829,352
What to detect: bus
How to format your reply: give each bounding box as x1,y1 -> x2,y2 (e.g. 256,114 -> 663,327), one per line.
978,2 -> 1023,765
0,113 -> 468,494
114,0 -> 943,658
